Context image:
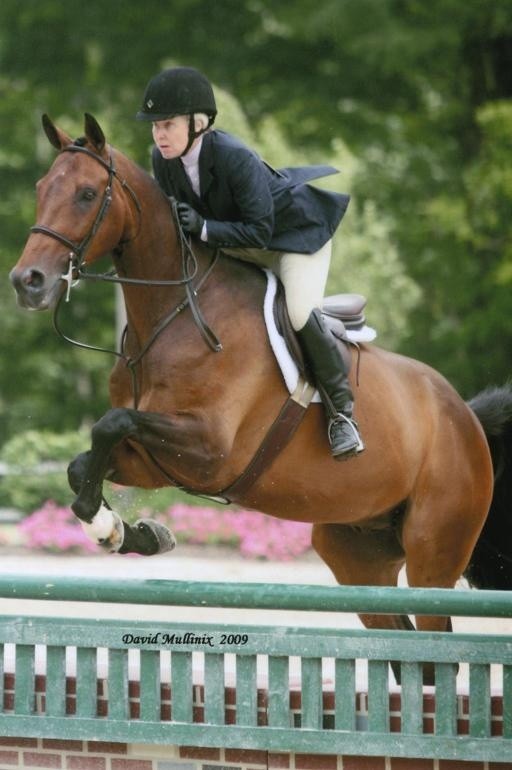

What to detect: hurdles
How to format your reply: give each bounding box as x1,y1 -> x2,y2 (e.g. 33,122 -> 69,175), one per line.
0,577 -> 512,762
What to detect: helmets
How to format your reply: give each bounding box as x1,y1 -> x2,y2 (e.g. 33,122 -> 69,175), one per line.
135,68 -> 217,122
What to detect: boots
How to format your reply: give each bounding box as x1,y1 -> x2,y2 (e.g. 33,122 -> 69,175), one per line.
294,308 -> 366,463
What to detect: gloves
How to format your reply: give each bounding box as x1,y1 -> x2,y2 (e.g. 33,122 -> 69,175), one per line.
178,202 -> 204,241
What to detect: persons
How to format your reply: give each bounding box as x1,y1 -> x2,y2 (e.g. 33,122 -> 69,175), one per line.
137,65 -> 363,461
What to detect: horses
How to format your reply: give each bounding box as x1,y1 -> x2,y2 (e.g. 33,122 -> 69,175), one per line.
8,112 -> 512,685
